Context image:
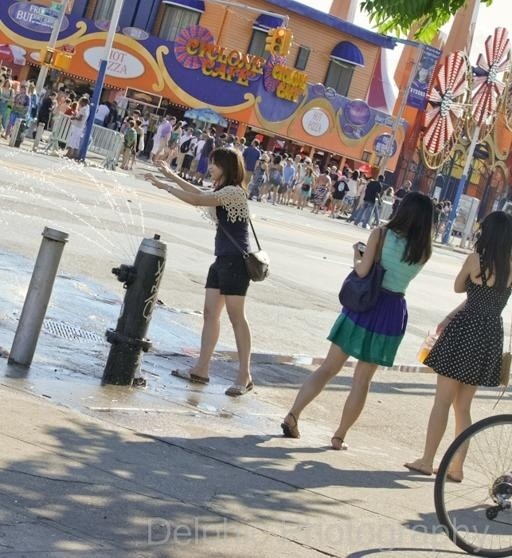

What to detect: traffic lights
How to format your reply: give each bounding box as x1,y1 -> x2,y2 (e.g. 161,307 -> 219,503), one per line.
40,47 -> 57,65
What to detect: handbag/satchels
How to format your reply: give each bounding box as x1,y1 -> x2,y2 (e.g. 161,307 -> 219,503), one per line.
302,184 -> 310,191
338,261 -> 386,314
244,248 -> 270,281
500,352 -> 511,387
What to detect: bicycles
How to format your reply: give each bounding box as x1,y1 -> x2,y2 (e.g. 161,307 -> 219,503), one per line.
430,411 -> 512,558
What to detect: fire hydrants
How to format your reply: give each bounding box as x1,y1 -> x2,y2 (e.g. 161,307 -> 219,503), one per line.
100,229 -> 174,385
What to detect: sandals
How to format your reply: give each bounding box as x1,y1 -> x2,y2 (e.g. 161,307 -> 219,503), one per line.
281,413 -> 301,438
331,436 -> 348,450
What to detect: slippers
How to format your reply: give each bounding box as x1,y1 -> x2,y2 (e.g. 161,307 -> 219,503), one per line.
433,469 -> 464,482
404,459 -> 434,475
224,382 -> 253,398
170,368 -> 209,385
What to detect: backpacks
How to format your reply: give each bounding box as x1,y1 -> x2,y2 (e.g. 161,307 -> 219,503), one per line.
180,137 -> 194,153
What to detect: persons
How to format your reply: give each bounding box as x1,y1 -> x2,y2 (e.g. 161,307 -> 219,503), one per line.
403,210 -> 512,485
143,146 -> 254,397
1,61 -> 452,248
280,191 -> 435,450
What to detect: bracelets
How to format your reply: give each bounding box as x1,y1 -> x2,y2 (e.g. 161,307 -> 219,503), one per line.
446,316 -> 453,321
